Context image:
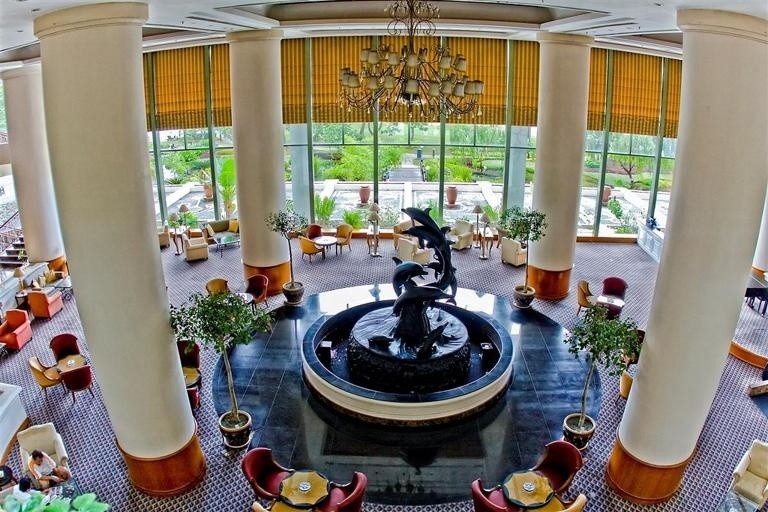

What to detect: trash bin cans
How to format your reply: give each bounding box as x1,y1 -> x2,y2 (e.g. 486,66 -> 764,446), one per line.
416,149 -> 422,159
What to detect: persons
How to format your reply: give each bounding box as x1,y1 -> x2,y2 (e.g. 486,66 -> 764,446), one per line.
13,479 -> 34,507
28,449 -> 68,489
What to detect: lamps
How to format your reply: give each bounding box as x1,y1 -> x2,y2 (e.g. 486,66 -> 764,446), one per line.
366,203 -> 381,257
177,203 -> 190,226
472,204 -> 494,260
332,0 -> 484,125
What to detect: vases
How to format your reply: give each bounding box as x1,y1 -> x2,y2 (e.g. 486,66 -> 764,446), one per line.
446,184 -> 458,205
202,180 -> 212,199
359,183 -> 370,203
602,184 -> 612,204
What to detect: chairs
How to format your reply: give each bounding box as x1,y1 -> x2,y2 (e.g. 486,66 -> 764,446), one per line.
177,340 -> 202,417
27,333 -> 94,403
205,274 -> 268,316
471,440 -> 587,512
241,447 -> 368,512
616,329 -> 645,403
298,222 -> 353,263
577,276 -> 628,319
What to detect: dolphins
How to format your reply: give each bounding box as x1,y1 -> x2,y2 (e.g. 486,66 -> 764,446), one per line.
392,208 -> 457,316
416,321 -> 449,358
368,336 -> 394,348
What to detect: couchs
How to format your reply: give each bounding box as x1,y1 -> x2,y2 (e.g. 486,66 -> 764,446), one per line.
158,218 -> 241,263
496,226 -> 528,268
0,262 -> 73,361
393,214 -> 438,266
446,218 -> 474,251
723,439 -> 768,512
0,422 -> 72,512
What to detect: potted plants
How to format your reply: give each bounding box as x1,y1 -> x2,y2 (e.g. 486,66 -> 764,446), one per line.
264,198 -> 310,305
563,304 -> 642,451
169,289 -> 276,448
498,205 -> 547,307
